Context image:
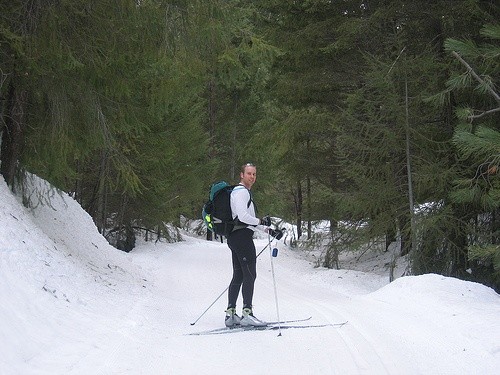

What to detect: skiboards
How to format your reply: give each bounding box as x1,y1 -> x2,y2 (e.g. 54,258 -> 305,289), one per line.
188,321 -> 349,335
210,316 -> 312,332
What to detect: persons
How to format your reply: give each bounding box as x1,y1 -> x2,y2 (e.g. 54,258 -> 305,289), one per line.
224,162 -> 283,328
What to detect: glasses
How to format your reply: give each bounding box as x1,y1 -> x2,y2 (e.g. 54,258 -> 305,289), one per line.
244,163 -> 255,166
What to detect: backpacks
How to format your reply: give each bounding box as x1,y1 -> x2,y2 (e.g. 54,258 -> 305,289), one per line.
202,181 -> 251,235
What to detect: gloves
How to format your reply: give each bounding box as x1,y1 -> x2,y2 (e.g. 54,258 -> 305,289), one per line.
269,228 -> 283,240
261,214 -> 271,227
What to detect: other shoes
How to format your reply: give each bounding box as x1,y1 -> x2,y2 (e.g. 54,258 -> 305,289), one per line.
225,307 -> 268,327
